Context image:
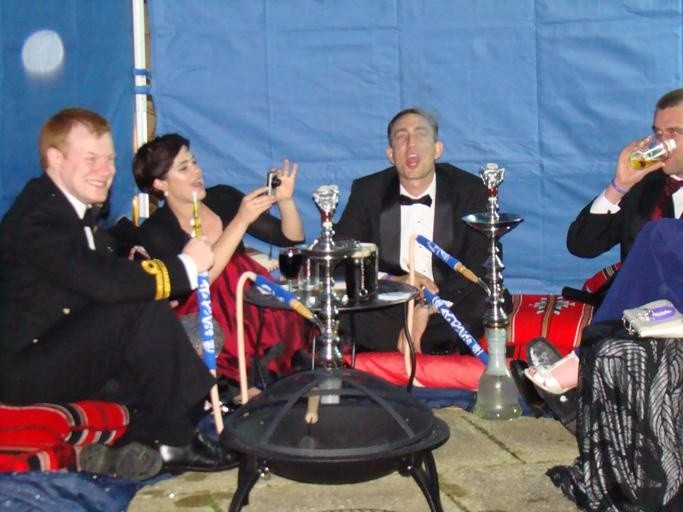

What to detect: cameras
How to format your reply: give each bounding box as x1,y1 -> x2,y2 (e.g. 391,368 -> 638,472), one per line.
266,172 -> 281,196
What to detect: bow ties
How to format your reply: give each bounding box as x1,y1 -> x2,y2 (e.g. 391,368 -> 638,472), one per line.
398,195 -> 432,206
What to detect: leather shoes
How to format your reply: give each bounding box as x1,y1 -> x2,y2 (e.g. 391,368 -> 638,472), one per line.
155,428 -> 242,478
79,442 -> 165,483
509,338 -> 578,438
428,340 -> 459,355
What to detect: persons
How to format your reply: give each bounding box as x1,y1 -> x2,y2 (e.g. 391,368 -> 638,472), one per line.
325,107 -> 513,359
126,131 -> 306,403
0,108 -> 239,472
563,89 -> 682,263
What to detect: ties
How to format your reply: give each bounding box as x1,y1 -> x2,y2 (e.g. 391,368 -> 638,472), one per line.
582,176 -> 683,294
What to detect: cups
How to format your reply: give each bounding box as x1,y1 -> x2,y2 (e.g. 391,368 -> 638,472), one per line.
628,130 -> 677,170
342,240 -> 383,302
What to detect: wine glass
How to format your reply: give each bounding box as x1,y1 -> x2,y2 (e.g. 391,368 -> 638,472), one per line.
276,248 -> 304,301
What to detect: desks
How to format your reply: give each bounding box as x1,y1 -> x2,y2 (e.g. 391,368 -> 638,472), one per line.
218,280 -> 451,398
